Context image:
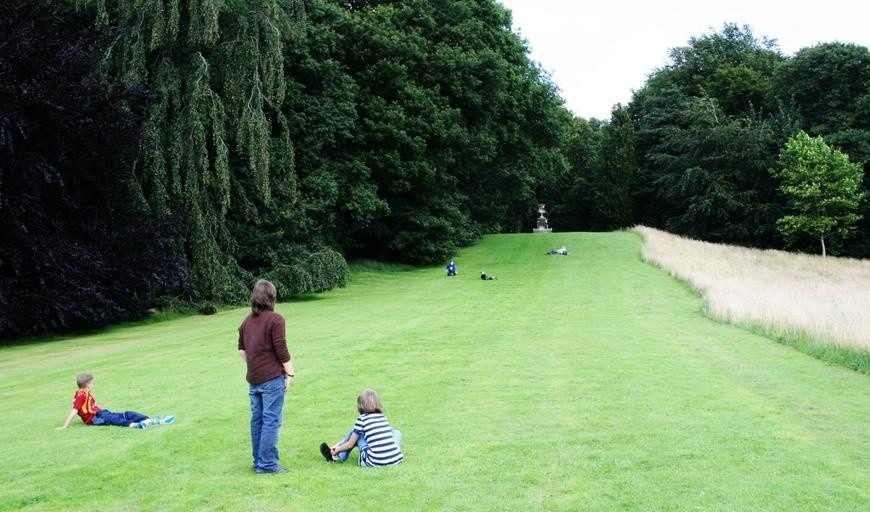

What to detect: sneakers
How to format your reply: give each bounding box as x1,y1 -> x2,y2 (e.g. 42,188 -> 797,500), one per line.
256,466 -> 287,474
140,421 -> 150,428
321,443 -> 338,462
161,417 -> 176,425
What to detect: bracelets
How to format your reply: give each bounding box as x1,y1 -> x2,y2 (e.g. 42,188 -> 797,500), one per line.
286,372 -> 294,377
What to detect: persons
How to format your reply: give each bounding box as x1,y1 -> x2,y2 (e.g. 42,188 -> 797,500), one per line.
320,388 -> 404,468
480,271 -> 497,280
238,279 -> 294,474
54,373 -> 176,430
545,246 -> 568,255
445,260 -> 457,276
